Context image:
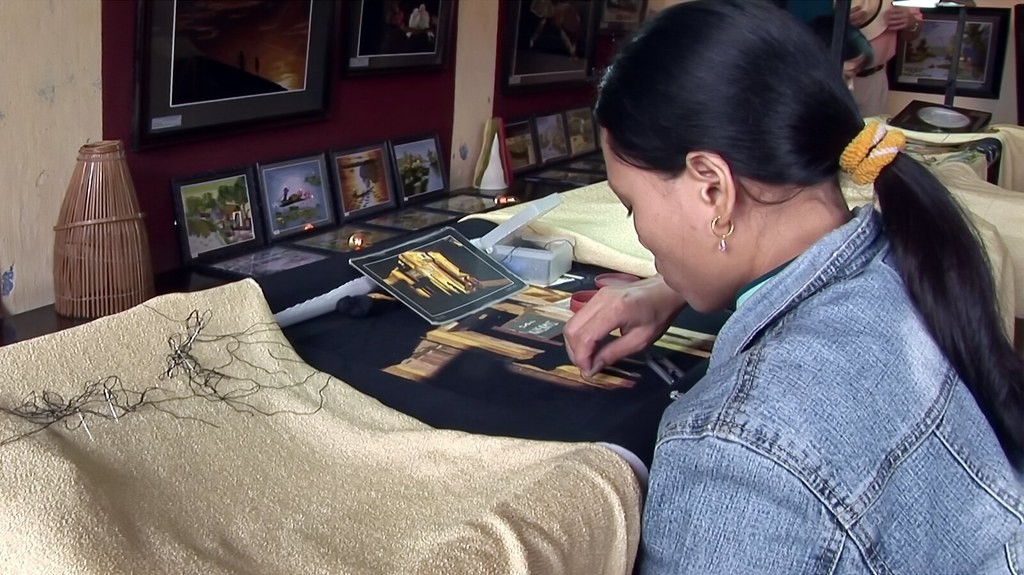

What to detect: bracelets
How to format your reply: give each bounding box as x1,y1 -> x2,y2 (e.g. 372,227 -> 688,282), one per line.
909,21 -> 920,33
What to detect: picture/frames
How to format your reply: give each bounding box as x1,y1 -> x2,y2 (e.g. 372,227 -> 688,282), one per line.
503,121 -> 536,174
888,6 -> 1011,100
595,0 -> 648,33
506,0 -> 591,86
533,111 -> 570,167
341,0 -> 452,79
170,163 -> 264,264
254,151 -> 338,242
131,0 -> 332,154
327,140 -> 400,224
389,132 -> 448,210
565,106 -> 597,156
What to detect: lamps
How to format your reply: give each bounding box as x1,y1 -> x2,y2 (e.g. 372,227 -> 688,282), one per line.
54,136 -> 152,317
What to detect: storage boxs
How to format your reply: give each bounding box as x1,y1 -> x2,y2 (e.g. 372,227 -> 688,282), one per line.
478,193 -> 575,286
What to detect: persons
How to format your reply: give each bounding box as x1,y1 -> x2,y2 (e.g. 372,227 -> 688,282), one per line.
843,0 -> 923,117
562,0 -> 1024,574
